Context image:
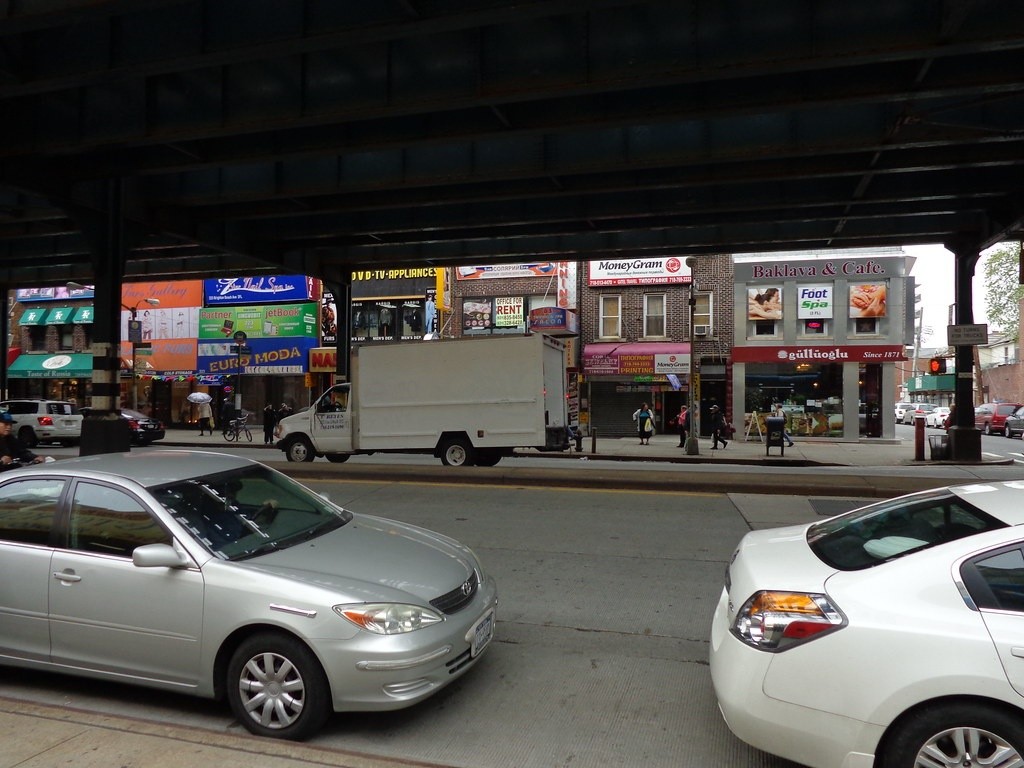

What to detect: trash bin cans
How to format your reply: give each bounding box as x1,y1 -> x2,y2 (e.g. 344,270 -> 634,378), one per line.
928,435 -> 950,460
766,417 -> 785,447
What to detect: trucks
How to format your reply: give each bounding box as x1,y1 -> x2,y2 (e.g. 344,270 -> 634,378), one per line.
272,332 -> 571,467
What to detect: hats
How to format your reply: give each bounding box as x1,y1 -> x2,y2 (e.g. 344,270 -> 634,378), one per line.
0,413 -> 18,424
710,405 -> 718,409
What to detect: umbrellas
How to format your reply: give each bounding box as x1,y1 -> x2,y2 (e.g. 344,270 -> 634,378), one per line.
188,392 -> 212,408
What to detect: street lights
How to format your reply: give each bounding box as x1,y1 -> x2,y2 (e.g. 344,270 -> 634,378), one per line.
685,256 -> 700,455
67,282 -> 160,410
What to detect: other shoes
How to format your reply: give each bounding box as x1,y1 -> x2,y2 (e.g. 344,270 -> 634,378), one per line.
639,442 -> 644,445
711,447 -> 717,449
724,442 -> 727,449
787,442 -> 793,447
646,442 -> 650,445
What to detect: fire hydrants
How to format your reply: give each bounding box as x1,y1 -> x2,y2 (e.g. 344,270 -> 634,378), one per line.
574,429 -> 584,452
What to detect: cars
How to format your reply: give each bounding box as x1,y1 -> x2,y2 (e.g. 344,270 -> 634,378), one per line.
75,406 -> 166,447
1004,405 -> 1024,439
903,401 -> 937,426
707,477 -> 1023,768
895,402 -> 912,423
926,406 -> 951,428
0,450 -> 503,738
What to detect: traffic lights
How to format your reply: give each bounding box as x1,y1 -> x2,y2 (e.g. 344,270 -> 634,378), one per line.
930,358 -> 946,373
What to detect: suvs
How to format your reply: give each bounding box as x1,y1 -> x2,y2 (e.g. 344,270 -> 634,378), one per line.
974,403 -> 1023,435
0,399 -> 84,448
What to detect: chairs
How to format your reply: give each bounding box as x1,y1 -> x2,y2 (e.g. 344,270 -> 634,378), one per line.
186,480 -> 226,513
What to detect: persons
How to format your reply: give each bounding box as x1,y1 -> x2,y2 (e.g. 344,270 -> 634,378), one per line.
198,398 -> 213,436
849,285 -> 885,318
221,397 -> 234,435
203,478 -> 280,551
379,308 -> 392,328
710,405 -> 728,449
0,410 -> 45,473
262,402 -> 292,444
410,308 -> 422,332
352,309 -> 367,331
677,403 -> 698,451
426,295 -> 434,333
942,404 -> 955,434
749,288 -> 782,318
632,403 -> 654,446
774,403 -> 794,447
140,309 -> 185,339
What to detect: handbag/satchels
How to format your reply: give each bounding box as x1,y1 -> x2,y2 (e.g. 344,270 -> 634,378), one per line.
783,412 -> 787,424
711,430 -> 720,444
210,417 -> 215,428
272,426 -> 280,438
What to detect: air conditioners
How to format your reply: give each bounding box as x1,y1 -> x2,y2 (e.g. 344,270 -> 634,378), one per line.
694,325 -> 707,336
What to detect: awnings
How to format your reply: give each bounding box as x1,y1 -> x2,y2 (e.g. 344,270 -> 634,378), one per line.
6,353 -> 95,379
19,305 -> 95,327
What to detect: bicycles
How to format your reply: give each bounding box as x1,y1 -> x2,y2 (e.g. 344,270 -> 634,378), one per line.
223,415 -> 252,442
